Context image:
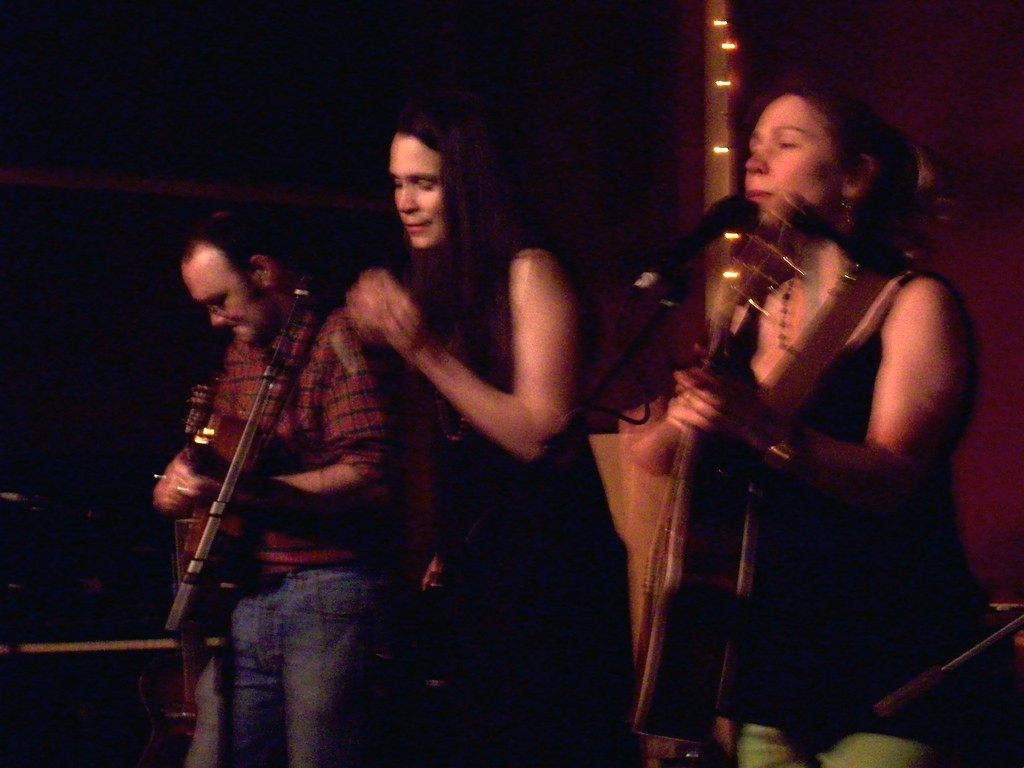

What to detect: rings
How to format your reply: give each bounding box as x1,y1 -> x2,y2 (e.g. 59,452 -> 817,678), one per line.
176,486 -> 181,495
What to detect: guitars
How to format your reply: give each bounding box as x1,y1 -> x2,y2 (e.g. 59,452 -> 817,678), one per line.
623,194 -> 802,742
136,393 -> 252,733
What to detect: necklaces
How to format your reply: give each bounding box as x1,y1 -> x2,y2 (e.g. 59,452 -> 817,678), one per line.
780,255 -> 853,350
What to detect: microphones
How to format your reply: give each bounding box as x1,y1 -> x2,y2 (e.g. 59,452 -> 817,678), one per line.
636,193 -> 759,291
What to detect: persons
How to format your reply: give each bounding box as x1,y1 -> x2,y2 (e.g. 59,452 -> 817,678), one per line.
153,73 -> 985,767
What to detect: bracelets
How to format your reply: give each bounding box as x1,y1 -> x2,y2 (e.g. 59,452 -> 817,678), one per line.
762,440 -> 798,469
